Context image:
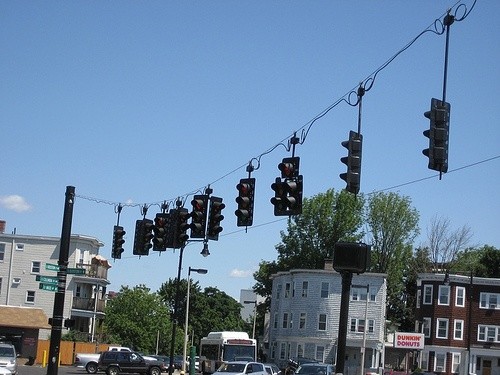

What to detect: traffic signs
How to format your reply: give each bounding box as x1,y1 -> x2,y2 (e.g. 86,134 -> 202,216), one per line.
67,269 -> 85,274
43,285 -> 57,291
46,263 -> 59,271
41,276 -> 58,283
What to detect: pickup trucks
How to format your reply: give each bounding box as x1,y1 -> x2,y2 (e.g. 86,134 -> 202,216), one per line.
74,346 -> 158,371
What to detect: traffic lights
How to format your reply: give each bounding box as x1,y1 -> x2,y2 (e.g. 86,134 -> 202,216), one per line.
208,196 -> 225,240
112,225 -> 126,258
191,195 -> 207,237
340,130 -> 363,194
422,98 -> 451,172
154,212 -> 169,250
270,156 -> 302,214
234,178 -> 255,225
137,219 -> 154,255
174,207 -> 190,247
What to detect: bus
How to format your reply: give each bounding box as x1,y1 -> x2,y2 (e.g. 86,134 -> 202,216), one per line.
199,331 -> 257,371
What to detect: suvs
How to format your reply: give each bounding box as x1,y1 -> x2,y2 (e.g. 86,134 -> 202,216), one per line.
295,365 -> 332,374
99,350 -> 163,375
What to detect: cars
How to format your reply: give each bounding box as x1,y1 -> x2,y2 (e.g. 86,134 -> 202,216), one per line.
211,361 -> 267,374
264,365 -> 274,374
265,362 -> 281,374
173,356 -> 183,367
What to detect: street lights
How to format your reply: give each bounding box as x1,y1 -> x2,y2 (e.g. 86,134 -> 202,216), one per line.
244,300 -> 257,339
444,270 -> 473,374
180,266 -> 207,373
353,284 -> 370,375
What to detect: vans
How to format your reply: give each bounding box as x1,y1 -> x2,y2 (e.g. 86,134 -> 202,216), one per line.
0,342 -> 18,375
150,355 -> 175,372
186,355 -> 200,370
